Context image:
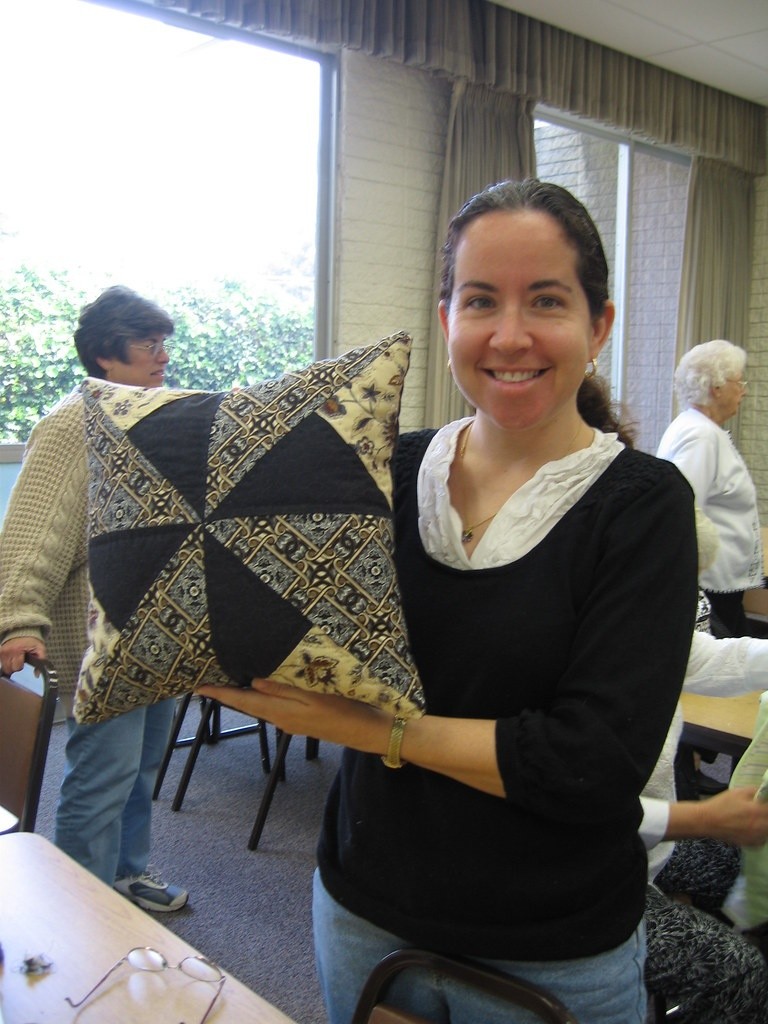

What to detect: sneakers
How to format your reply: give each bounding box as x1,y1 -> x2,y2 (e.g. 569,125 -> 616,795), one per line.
113,871 -> 190,912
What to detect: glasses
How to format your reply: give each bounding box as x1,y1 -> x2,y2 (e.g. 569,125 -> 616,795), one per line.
65,947 -> 226,1024
726,380 -> 748,391
129,343 -> 175,357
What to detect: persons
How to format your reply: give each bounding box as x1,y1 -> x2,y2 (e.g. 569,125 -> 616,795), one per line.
657,338 -> 765,640
182,175 -> 701,1024
640,623 -> 768,1024
2,284 -> 193,915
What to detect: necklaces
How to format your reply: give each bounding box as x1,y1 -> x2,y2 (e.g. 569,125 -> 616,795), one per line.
454,418 -> 500,543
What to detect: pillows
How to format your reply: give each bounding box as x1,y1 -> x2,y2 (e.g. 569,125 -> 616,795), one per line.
72,329 -> 427,727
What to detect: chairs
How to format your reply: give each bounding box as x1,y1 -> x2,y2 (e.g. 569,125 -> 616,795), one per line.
349,947 -> 582,1024
0,653 -> 60,834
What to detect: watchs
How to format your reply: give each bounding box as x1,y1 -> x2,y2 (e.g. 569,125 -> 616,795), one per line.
379,712 -> 412,768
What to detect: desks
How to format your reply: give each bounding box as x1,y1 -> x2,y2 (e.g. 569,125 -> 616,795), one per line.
675,684 -> 768,758
0,803 -> 302,1024
